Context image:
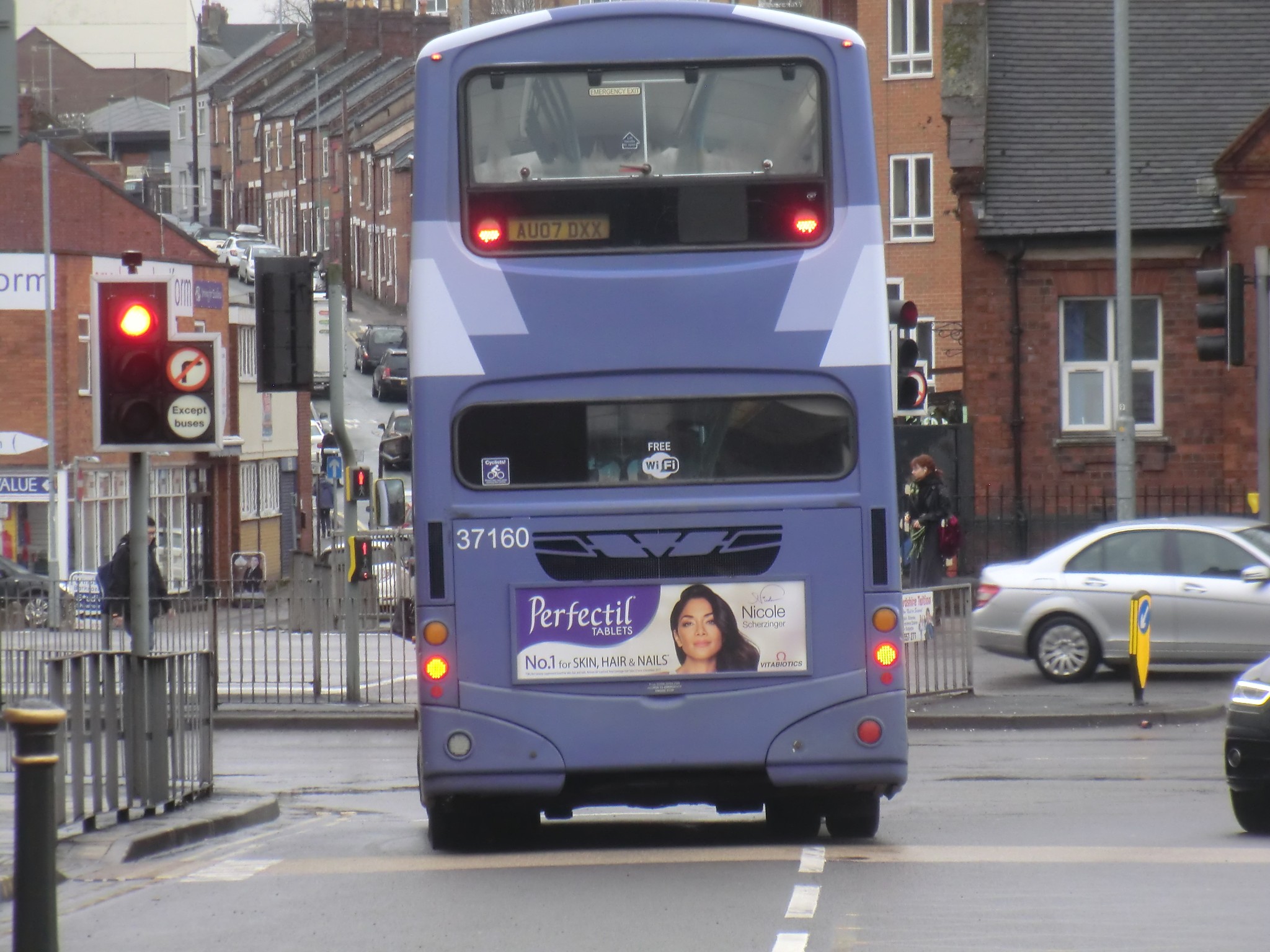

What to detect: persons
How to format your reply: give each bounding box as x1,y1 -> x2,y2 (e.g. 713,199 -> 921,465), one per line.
904,454 -> 951,629
312,471 -> 334,538
924,608 -> 934,639
243,555 -> 262,594
649,584 -> 760,674
321,429 -> 343,488
112,515 -> 176,657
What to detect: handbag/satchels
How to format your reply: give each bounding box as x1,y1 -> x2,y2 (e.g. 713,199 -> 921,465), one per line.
940,512 -> 964,559
95,541 -> 127,614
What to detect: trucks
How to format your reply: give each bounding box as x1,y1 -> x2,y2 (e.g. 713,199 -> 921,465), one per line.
313,292 -> 347,387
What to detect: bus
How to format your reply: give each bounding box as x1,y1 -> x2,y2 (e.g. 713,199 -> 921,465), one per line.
409,0 -> 909,846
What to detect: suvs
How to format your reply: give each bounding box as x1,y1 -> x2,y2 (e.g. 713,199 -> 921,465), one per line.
373,348 -> 408,401
355,324 -> 406,374
215,234 -> 265,274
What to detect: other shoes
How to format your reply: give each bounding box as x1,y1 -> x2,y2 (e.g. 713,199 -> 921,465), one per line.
321,534 -> 325,537
327,532 -> 331,536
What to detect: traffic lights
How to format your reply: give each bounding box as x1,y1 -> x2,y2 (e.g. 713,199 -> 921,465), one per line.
348,536 -> 372,581
889,298 -> 918,409
346,466 -> 369,502
1196,250 -> 1244,372
91,275 -> 166,451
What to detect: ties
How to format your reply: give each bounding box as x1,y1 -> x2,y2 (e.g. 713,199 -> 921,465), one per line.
248,568 -> 253,577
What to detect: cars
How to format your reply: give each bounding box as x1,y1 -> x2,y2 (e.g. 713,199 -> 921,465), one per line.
970,515 -> 1270,683
315,490 -> 414,628
313,266 -> 327,293
0,556 -> 75,628
193,227 -> 233,254
377,408 -> 412,468
237,245 -> 283,285
311,420 -> 326,465
1225,659 -> 1270,831
158,213 -> 204,237
